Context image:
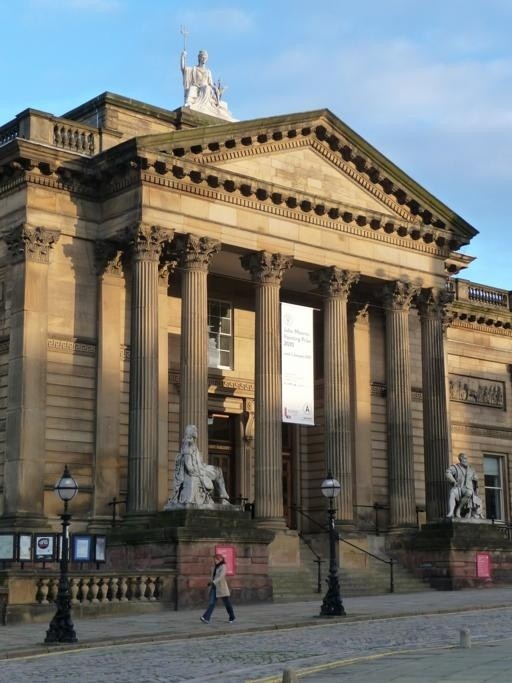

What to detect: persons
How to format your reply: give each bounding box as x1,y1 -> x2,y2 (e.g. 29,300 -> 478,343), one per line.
181,49 -> 239,122
200,554 -> 237,624
179,424 -> 234,505
445,453 -> 486,519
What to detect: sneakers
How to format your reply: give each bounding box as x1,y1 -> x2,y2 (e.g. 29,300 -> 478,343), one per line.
200,617 -> 236,624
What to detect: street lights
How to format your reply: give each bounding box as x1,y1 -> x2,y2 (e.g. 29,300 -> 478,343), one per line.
46,464 -> 78,645
318,468 -> 346,615
